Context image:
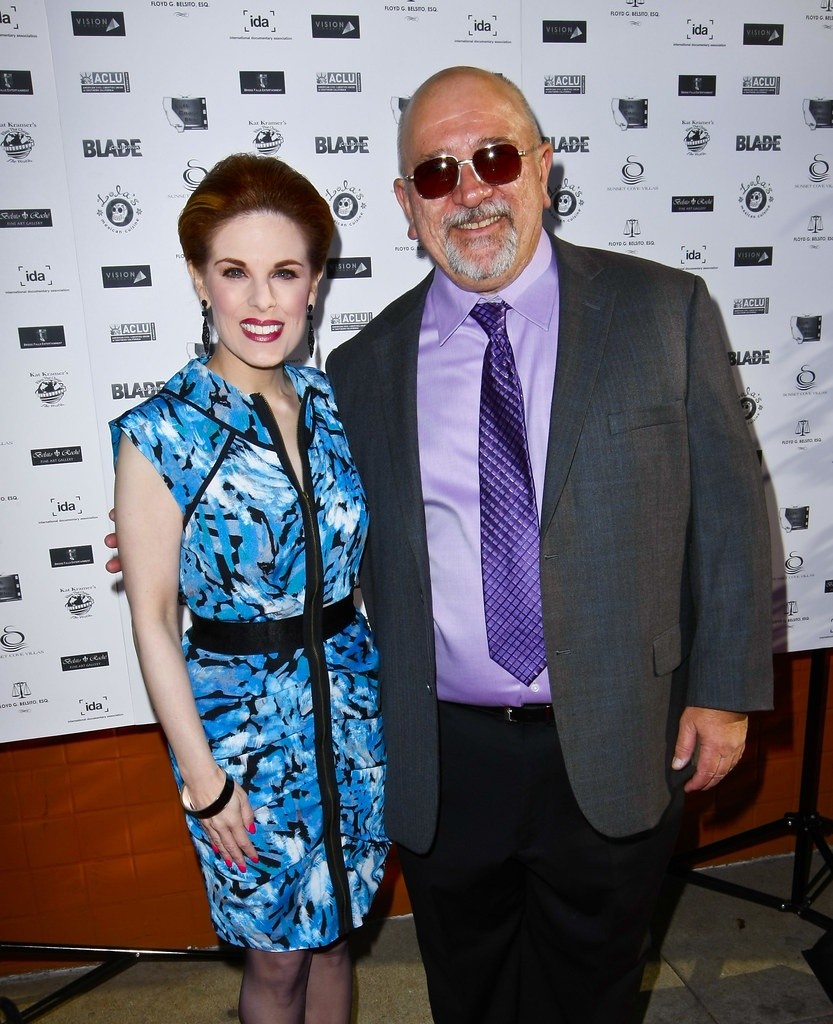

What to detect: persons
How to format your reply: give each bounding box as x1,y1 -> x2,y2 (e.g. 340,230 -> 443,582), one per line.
104,65 -> 778,1024
101,152 -> 396,1024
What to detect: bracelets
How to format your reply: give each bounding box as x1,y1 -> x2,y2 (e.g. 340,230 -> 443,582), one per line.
179,764 -> 234,819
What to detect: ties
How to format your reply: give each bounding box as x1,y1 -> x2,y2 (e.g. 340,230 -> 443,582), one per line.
469,302 -> 547,687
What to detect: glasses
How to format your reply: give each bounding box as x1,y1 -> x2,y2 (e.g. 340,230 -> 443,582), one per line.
399,144 -> 537,199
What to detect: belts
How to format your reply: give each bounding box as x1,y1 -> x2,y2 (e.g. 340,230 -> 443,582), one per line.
450,702 -> 556,725
185,593 -> 355,655
